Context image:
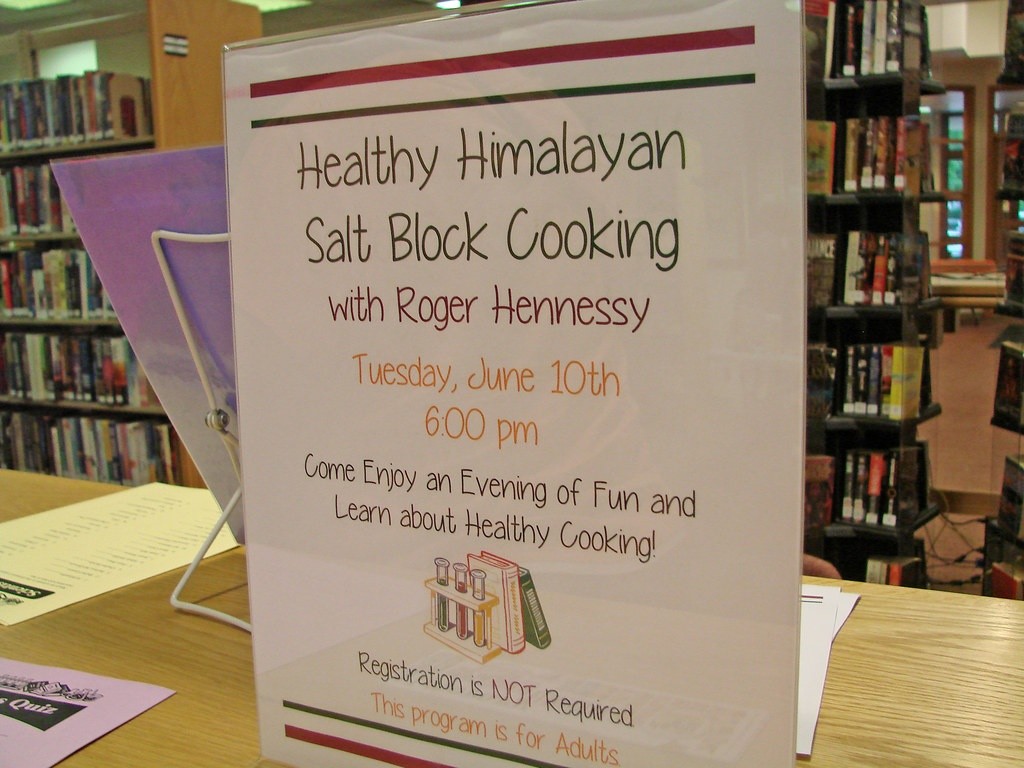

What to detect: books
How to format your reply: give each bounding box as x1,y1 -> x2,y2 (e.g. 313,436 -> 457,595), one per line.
804,0 -> 926,590
0,69 -> 184,487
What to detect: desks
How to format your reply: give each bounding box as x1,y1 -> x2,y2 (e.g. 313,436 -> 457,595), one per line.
931,272 -> 1008,332
0,469 -> 1024,768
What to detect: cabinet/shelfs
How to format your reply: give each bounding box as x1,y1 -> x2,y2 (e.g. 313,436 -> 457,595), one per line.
0,0 -> 263,490
799,0 -> 943,591
